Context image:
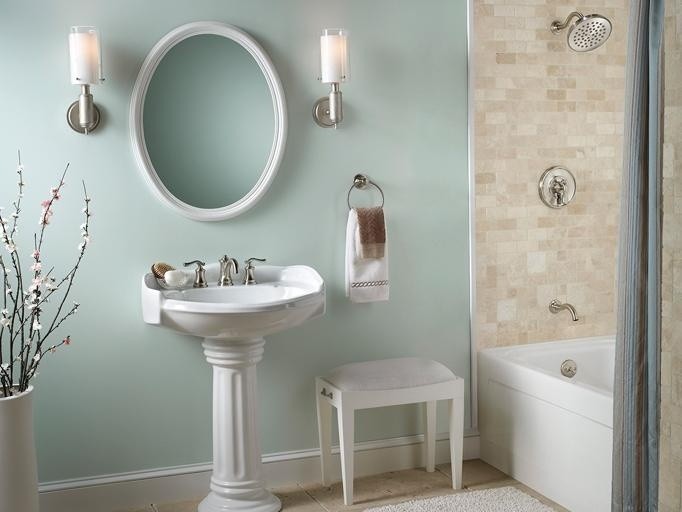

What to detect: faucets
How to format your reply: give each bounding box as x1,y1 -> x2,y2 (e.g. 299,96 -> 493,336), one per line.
218,255 -> 240,286
549,299 -> 579,321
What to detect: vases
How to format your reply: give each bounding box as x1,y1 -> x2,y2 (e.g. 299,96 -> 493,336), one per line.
0,384 -> 44,512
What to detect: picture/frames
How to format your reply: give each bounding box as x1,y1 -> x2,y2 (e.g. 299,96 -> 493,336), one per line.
128,14 -> 287,225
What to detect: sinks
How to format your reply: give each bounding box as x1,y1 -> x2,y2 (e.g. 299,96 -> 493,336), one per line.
141,262 -> 326,338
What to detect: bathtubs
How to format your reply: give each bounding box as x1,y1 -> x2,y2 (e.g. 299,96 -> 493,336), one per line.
477,333 -> 617,512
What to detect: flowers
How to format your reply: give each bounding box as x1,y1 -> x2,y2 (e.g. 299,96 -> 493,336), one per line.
1,139 -> 92,394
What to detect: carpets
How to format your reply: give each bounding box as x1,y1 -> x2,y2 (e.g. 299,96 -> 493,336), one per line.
358,485 -> 556,511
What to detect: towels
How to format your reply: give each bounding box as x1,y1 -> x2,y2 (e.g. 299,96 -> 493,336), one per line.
341,206 -> 392,303
353,207 -> 387,260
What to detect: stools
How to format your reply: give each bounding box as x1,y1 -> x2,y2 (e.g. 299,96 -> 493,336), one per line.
311,352 -> 473,505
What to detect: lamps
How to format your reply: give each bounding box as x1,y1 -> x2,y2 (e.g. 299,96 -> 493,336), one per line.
315,21 -> 350,129
65,24 -> 107,133
